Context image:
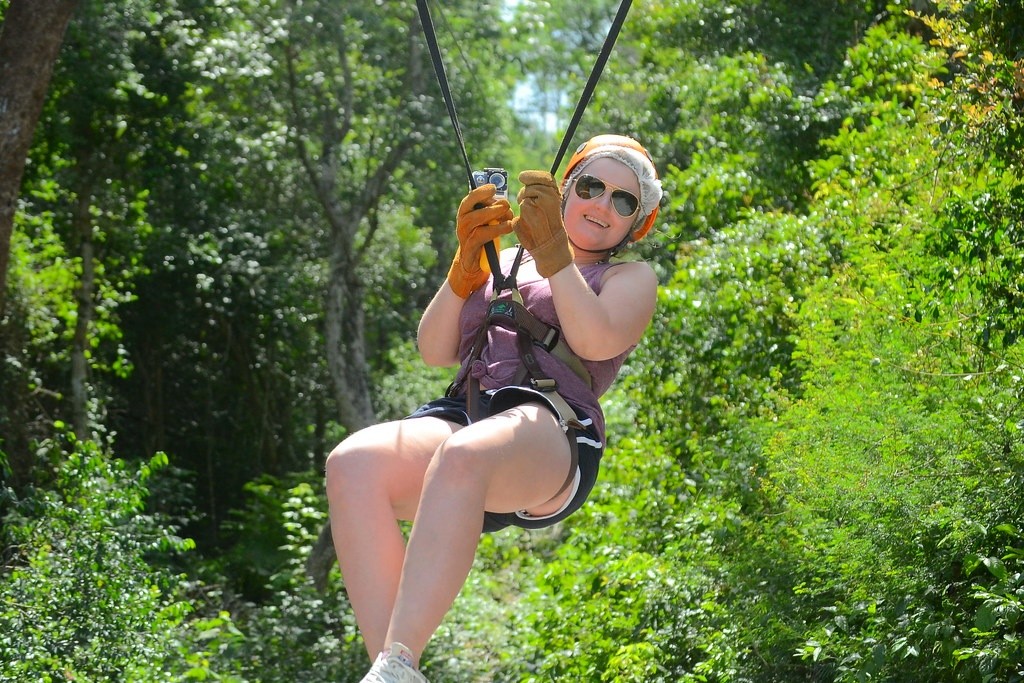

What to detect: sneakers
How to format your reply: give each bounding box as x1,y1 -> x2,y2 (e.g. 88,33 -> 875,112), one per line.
360,642 -> 429,683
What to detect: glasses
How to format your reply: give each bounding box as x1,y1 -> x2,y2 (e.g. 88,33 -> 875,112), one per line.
573,174 -> 641,219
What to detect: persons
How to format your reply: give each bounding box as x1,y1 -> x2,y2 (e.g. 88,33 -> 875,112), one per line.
326,135 -> 663,683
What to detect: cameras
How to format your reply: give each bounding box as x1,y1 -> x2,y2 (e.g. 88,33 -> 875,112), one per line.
469,168 -> 508,200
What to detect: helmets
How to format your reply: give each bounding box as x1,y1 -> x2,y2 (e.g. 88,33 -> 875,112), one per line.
560,134 -> 663,243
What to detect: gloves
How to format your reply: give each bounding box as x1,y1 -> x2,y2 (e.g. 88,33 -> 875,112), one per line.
447,184 -> 515,299
511,170 -> 575,278
